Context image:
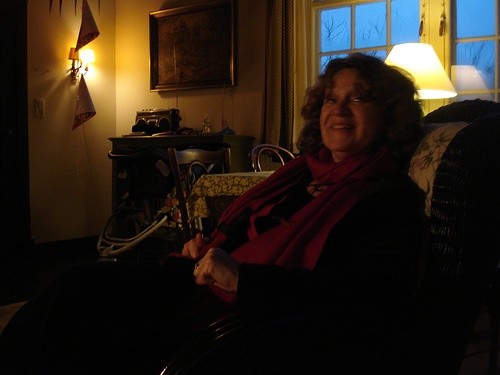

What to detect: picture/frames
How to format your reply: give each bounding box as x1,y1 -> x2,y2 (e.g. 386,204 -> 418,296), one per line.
149,0 -> 238,92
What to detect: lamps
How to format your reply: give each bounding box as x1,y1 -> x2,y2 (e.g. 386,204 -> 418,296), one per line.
449,64 -> 489,101
68,47 -> 94,81
384,42 -> 458,100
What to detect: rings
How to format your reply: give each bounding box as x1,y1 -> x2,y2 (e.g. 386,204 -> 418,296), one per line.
194,263 -> 199,268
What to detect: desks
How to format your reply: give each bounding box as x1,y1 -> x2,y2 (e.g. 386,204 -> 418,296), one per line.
107,127 -> 236,235
188,169 -> 274,232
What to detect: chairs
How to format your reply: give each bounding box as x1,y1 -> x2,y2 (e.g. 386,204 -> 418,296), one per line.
167,144 -> 296,243
159,102 -> 500,375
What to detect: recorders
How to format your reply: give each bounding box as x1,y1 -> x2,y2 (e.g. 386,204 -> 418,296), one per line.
136,108 -> 179,132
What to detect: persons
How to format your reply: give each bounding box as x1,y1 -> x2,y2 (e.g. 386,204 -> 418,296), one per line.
0,53 -> 428,375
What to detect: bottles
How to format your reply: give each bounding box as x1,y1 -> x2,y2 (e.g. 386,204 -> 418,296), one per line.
203,115 -> 212,134
